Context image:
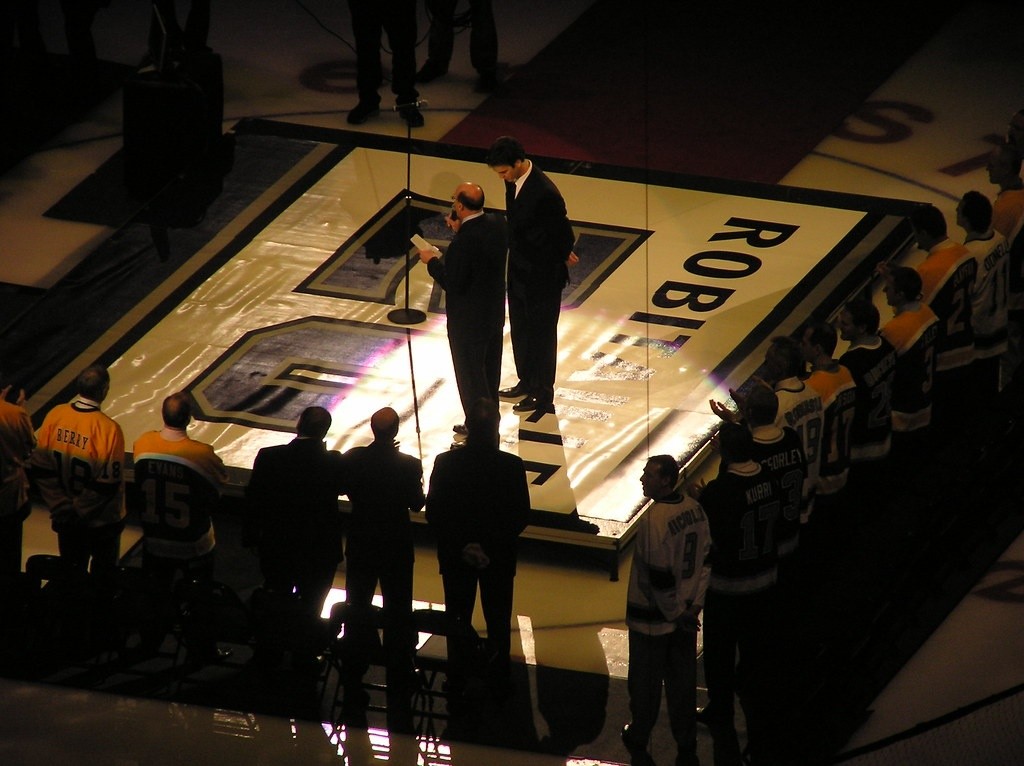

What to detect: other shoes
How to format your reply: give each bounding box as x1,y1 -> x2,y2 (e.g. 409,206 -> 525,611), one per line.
513,396 -> 552,412
622,722 -> 647,756
400,105 -> 425,127
450,443 -> 466,451
477,65 -> 498,94
695,707 -> 709,722
453,424 -> 468,435
498,380 -> 526,398
417,58 -> 451,81
347,100 -> 380,124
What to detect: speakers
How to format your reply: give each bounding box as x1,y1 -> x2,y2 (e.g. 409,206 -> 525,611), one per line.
123,52 -> 224,200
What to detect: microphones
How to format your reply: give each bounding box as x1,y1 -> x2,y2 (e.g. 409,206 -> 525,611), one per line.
451,203 -> 457,221
392,99 -> 429,112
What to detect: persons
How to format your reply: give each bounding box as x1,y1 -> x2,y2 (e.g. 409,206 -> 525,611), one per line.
0,0 -> 115,60
485,137 -> 580,409
687,110 -> 1024,766
425,416 -> 531,702
418,186 -> 510,447
0,362 -> 129,651
344,410 -> 425,734
416,0 -> 500,92
138,0 -> 237,228
237,406 -> 348,693
620,454 -> 713,757
345,0 -> 424,126
132,398 -> 235,657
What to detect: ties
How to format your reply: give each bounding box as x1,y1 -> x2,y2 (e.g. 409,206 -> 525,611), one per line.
512,184 -> 516,200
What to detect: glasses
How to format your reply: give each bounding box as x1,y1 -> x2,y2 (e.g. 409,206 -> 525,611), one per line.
452,196 -> 459,203
